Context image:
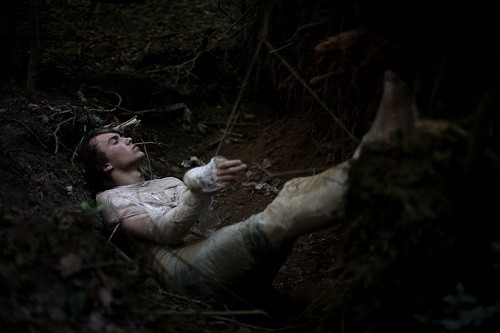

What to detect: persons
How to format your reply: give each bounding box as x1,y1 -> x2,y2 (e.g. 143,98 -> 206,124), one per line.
80,69 -> 423,308
